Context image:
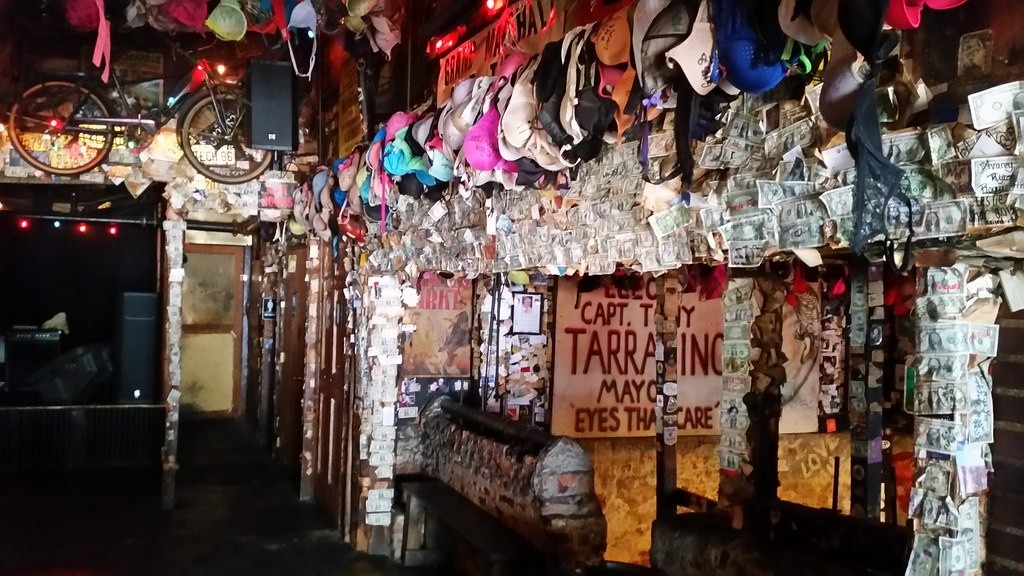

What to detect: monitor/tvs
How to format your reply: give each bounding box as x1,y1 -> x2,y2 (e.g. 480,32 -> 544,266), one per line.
0,211 -> 158,338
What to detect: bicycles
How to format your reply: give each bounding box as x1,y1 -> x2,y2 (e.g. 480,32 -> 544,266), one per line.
8,26 -> 274,184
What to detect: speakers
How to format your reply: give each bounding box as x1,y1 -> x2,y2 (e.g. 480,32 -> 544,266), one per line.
245,58 -> 299,152
119,290 -> 163,405
